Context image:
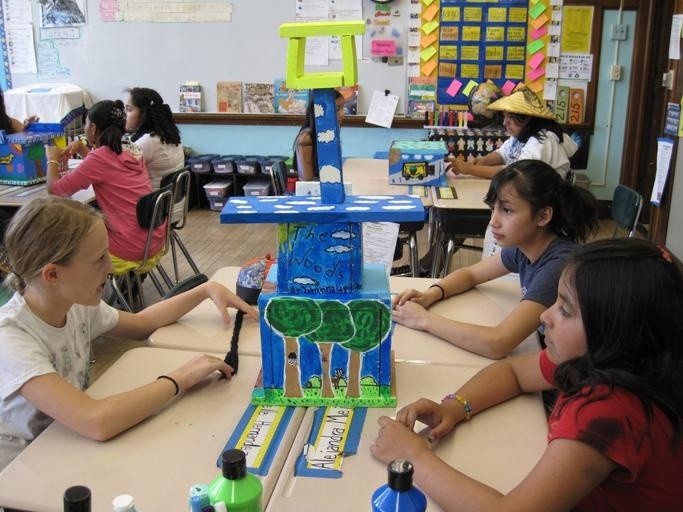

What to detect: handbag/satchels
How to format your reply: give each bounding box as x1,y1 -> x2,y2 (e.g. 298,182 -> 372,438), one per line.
292,122 -> 317,181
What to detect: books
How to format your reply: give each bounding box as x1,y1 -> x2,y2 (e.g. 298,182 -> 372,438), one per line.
407,76 -> 437,120
216,79 -> 310,115
180,84 -> 202,114
336,85 -> 359,116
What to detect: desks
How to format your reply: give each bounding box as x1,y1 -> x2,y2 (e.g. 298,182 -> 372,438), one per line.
432,162 -> 495,279
342,157 -> 433,278
1,158 -> 98,208
147,268 -> 265,356
1,346 -> 306,510
388,276 -> 543,367
264,364 -> 550,512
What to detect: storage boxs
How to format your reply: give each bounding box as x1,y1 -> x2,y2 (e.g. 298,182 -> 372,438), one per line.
184,153 -> 290,212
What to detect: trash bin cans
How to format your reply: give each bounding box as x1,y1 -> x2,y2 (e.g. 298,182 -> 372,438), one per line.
575,173 -> 593,192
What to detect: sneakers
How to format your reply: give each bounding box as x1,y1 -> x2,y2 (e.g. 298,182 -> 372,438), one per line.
116,272 -> 147,304
390,264 -> 431,277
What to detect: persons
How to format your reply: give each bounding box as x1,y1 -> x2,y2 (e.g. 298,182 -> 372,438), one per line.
0,197 -> 261,472
116,87 -> 185,304
292,89 -> 345,181
44,100 -> 168,275
416,85 -> 571,276
0,87 -> 40,133
370,243 -> 683,511
391,160 -> 601,360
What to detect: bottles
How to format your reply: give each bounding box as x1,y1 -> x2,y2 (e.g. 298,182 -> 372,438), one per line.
112,494 -> 139,512
370,458 -> 429,512
63,485 -> 92,512
207,447 -> 262,512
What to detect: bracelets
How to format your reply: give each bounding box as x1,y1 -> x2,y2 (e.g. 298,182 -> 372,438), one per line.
442,395 -> 472,422
157,375 -> 179,395
429,285 -> 444,301
47,160 -> 61,166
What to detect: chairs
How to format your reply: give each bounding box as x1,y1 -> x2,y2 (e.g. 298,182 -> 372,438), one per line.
440,167 -> 577,278
158,166 -> 200,286
607,182 -> 643,239
270,160 -> 296,197
108,184 -> 173,314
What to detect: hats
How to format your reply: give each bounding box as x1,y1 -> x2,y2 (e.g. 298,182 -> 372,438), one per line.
487,84 -> 556,121
236,253 -> 277,305
